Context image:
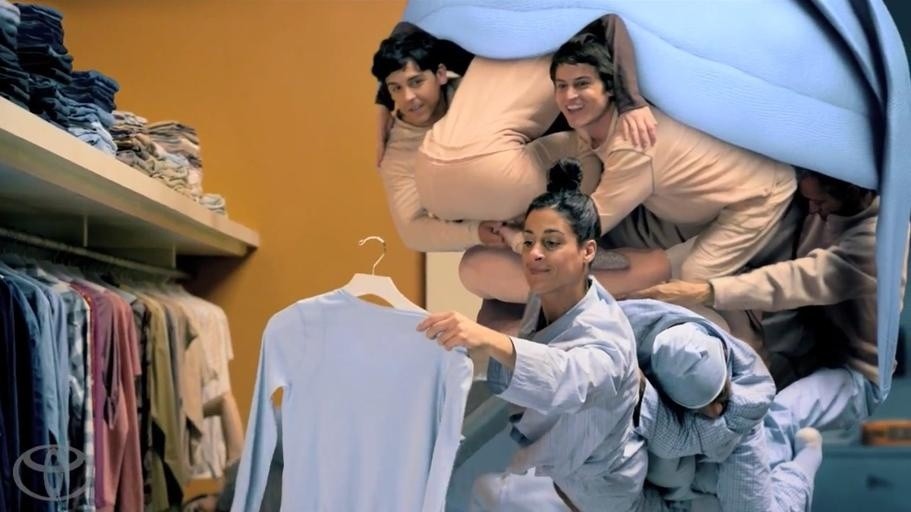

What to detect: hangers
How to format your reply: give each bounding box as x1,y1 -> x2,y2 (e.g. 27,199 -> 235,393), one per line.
0,227 -> 192,280
342,235 -> 432,313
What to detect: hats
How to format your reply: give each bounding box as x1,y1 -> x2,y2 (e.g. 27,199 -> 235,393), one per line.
649,322 -> 729,410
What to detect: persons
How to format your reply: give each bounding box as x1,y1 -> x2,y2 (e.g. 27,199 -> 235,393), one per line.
371,15 -> 911,509
